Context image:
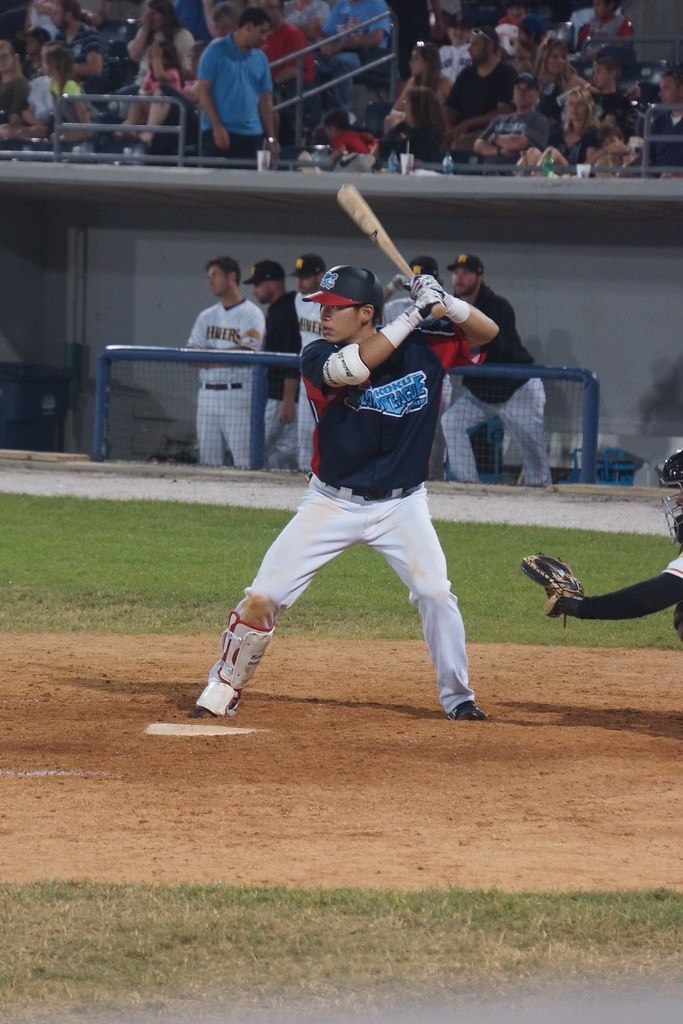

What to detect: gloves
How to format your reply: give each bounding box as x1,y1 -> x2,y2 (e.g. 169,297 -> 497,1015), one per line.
388,274 -> 407,291
379,274 -> 470,349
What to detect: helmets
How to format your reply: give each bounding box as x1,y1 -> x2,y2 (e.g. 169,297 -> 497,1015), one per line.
660,449 -> 683,543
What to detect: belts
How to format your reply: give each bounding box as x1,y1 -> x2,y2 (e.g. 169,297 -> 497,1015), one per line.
318,476 -> 412,502
201,383 -> 242,390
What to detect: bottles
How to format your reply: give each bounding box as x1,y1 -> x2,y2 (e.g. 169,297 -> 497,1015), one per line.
443,153 -> 454,175
543,150 -> 554,176
633,462 -> 660,487
519,151 -> 530,176
388,150 -> 398,174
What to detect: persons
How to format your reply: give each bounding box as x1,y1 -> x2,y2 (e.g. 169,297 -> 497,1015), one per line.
520,449 -> 683,645
195,263 -> 500,721
186,256 -> 553,487
0,0 -> 683,178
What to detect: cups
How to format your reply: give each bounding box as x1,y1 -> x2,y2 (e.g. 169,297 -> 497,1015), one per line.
400,154 -> 414,174
257,150 -> 271,170
557,85 -> 582,106
577,164 -> 591,178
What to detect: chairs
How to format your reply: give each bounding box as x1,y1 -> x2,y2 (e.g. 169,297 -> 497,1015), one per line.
0,0 -> 676,174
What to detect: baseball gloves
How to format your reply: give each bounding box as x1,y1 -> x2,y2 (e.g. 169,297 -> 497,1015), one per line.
520,549 -> 586,630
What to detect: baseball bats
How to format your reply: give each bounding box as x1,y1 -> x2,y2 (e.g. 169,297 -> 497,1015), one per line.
334,182 -> 448,319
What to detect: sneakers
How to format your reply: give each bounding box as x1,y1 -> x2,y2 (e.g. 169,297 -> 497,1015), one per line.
191,694 -> 239,719
448,702 -> 486,721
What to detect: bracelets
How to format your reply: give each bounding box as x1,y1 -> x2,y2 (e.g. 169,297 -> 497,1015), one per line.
267,136 -> 275,143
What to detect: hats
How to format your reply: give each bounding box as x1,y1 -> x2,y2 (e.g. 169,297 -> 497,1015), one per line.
404,255 -> 443,291
514,72 -> 539,89
243,259 -> 285,284
520,14 -> 547,43
446,254 -> 484,275
302,265 -> 384,312
285,254 -> 326,276
